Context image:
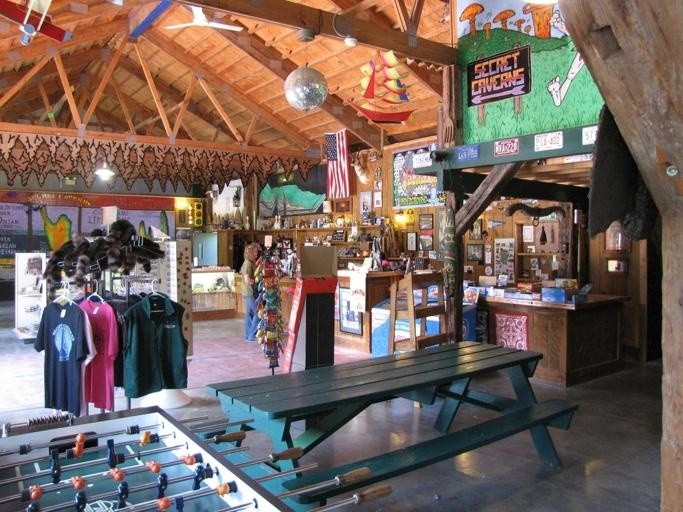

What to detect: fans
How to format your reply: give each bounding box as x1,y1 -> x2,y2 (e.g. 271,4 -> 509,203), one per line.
164,5 -> 243,35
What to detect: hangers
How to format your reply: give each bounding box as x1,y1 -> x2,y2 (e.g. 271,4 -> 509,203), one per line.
41,253 -> 162,419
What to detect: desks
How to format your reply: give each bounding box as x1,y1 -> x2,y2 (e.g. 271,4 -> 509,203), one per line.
191,264 -> 238,322
477,285 -> 632,388
338,268 -> 448,338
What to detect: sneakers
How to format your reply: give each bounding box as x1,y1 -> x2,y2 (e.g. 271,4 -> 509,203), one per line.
249,338 -> 260,343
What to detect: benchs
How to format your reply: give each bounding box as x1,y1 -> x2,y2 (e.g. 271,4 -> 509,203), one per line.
206,340 -> 580,507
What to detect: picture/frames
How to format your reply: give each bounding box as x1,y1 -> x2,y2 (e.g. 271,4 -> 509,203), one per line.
407,232 -> 417,252
465,265 -> 473,273
375,167 -> 382,177
467,242 -> 482,261
417,230 -> 433,252
428,250 -> 437,259
373,180 -> 384,190
469,219 -> 483,240
419,213 -> 433,231
373,192 -> 384,208
334,197 -> 353,213
359,190 -> 373,214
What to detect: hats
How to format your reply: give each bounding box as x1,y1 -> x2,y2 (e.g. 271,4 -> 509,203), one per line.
104,219 -> 136,245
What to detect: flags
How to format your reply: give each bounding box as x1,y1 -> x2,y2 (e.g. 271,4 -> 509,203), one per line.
324,128 -> 350,199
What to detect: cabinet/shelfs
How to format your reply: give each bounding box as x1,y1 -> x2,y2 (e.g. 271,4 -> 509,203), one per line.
513,219 -> 565,284
217,228 -> 412,270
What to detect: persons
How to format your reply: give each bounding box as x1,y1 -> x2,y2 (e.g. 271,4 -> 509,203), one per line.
240,245 -> 258,342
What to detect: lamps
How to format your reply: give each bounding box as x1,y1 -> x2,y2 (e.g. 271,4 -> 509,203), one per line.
95,161 -> 115,183
176,198 -> 189,211
393,211 -> 408,229
283,65 -> 329,113
